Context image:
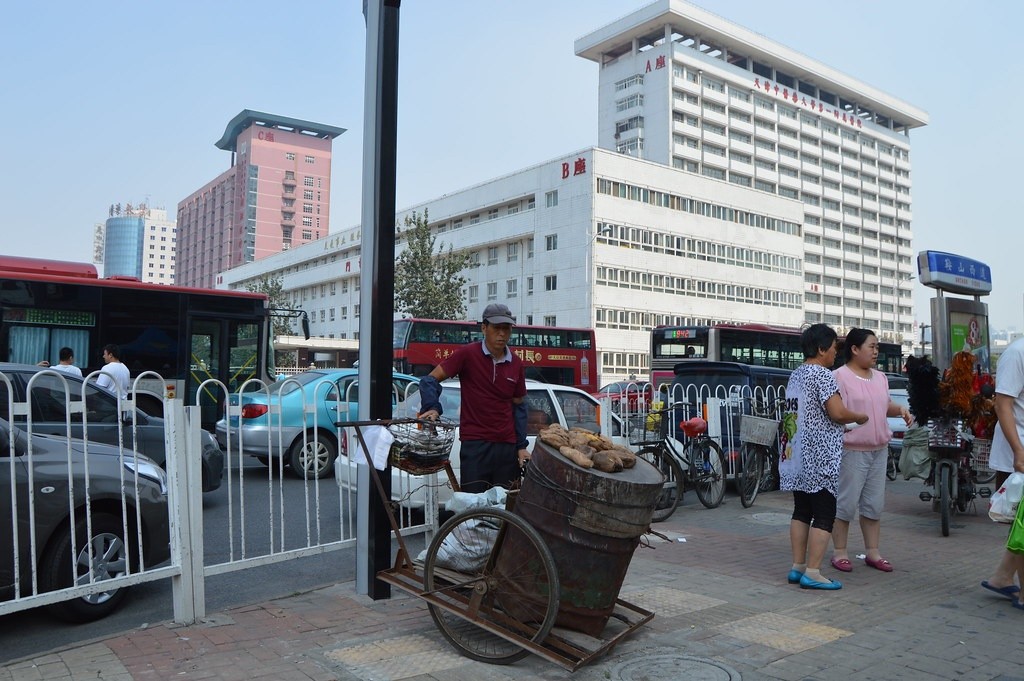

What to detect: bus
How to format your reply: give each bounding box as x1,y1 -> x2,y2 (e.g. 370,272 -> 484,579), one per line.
392,319 -> 598,394
649,323 -> 905,391
0,256 -> 311,452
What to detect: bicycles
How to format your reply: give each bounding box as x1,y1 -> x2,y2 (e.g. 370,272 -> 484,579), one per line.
630,402 -> 728,523
731,397 -> 786,508
885,444 -> 897,481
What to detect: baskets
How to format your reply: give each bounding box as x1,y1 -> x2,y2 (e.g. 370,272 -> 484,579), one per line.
627,412 -> 668,445
386,416 -> 456,475
972,438 -> 992,472
739,414 -> 781,447
926,417 -> 968,449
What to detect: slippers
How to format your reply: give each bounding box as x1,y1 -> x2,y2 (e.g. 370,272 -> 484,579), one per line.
981,581 -> 1024,610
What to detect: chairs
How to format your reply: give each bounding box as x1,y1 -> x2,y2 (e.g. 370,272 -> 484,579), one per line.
687,347 -> 696,355
732,353 -> 797,370
534,340 -> 553,347
526,410 -> 549,434
473,337 -> 478,342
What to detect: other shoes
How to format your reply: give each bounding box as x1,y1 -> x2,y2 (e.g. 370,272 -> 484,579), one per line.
831,555 -> 852,572
865,555 -> 893,572
788,569 -> 803,584
799,574 -> 843,590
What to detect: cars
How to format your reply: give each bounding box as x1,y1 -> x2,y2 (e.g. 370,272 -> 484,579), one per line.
0,419 -> 171,625
213,367 -> 419,480
334,380 -> 690,536
593,380 -> 652,413
844,389 -> 971,460
0,362 -> 224,491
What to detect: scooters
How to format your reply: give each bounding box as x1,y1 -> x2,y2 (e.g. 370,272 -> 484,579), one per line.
919,418 -> 996,536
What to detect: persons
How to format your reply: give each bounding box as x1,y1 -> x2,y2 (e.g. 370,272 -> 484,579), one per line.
37,361 -> 49,366
778,323 -> 869,590
95,344 -> 130,411
832,326 -> 915,572
980,335 -> 1024,608
276,373 -> 286,381
49,348 -> 82,395
418,304 -> 532,495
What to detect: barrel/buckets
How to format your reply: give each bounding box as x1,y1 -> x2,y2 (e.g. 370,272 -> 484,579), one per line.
490,434 -> 667,634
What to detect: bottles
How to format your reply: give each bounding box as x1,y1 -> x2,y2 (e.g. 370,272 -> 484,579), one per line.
580,351 -> 589,385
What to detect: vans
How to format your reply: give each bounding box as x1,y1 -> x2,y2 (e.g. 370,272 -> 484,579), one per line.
660,362 -> 794,493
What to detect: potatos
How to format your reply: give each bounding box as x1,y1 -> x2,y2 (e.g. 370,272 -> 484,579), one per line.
540,423 -> 636,473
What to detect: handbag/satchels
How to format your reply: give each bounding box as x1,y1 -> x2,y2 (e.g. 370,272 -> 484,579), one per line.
987,471 -> 1024,524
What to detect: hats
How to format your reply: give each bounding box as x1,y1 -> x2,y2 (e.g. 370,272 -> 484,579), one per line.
37,361 -> 50,367
482,304 -> 517,326
